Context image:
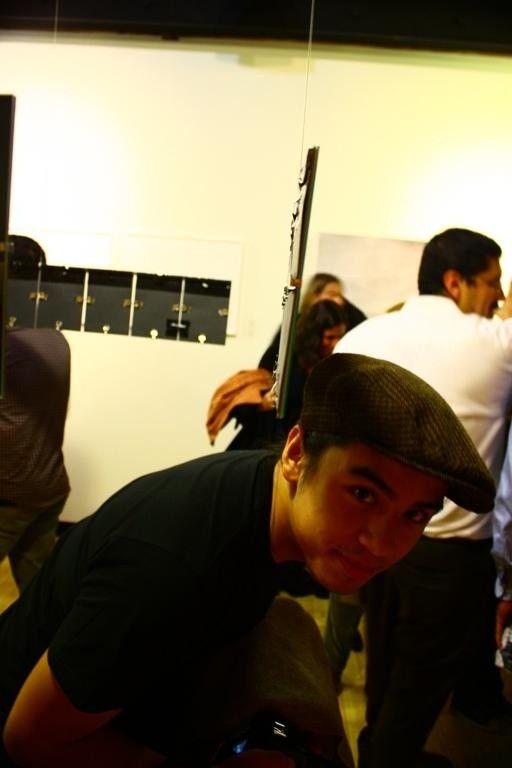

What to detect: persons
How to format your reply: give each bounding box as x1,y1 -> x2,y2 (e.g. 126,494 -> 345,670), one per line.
324,228 -> 511,767
0,353 -> 496,768
0,233 -> 71,590
259,273 -> 367,372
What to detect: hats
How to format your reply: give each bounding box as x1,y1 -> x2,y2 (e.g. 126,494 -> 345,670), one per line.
300,352 -> 497,514
